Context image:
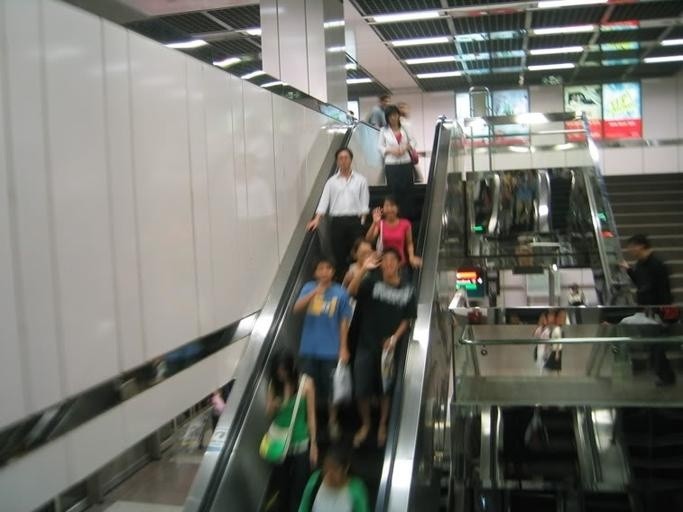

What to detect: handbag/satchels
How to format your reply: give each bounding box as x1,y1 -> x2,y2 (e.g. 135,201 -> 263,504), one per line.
407,145 -> 418,165
375,236 -> 383,253
257,419 -> 293,467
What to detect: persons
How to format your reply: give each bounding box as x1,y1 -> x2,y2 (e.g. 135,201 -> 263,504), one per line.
294,254 -> 354,442
530,311 -> 550,373
307,147 -> 371,269
375,105 -> 415,218
544,311 -> 569,374
567,282 -> 585,306
261,348 -> 318,512
365,92 -> 390,127
624,228 -> 679,385
340,236 -> 372,291
365,193 -> 424,270
297,443 -> 372,512
346,246 -> 417,449
397,100 -> 424,182
